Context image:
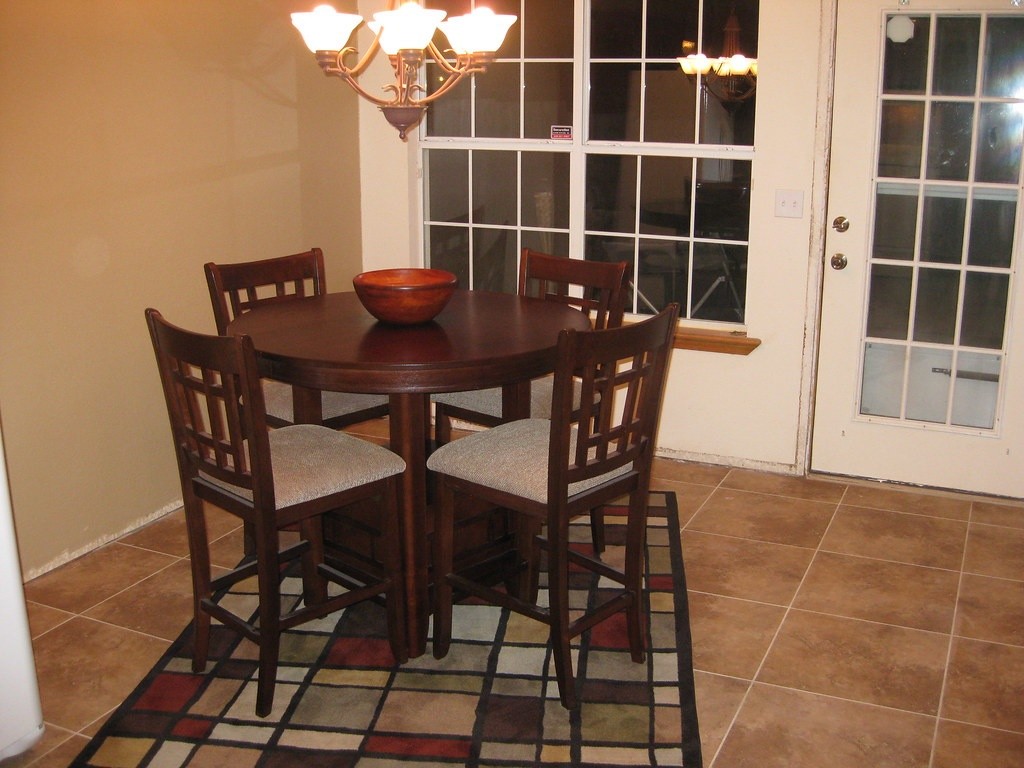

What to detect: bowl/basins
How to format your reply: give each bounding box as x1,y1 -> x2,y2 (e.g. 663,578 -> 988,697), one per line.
353,267 -> 458,325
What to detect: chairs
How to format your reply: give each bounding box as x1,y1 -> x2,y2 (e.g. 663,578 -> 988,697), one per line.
429,247 -> 632,554
202,246 -> 391,558
144,308 -> 410,715
428,302 -> 677,711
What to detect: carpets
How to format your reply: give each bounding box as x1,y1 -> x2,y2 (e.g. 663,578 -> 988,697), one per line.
60,489 -> 703,767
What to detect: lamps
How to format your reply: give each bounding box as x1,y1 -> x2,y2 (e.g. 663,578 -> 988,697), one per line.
289,0 -> 519,143
677,4 -> 760,105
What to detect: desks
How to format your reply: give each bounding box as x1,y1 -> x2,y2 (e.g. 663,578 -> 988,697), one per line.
636,202 -> 748,316
222,287 -> 593,660
